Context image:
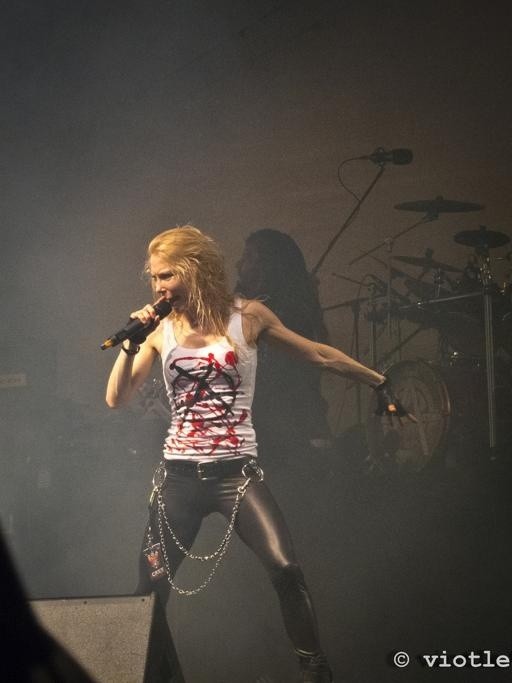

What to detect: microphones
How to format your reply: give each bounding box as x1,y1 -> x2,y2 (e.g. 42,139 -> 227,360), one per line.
361,146 -> 414,165
100,302 -> 172,352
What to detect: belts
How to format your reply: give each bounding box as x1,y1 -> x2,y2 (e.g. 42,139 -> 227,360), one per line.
167,459 -> 252,480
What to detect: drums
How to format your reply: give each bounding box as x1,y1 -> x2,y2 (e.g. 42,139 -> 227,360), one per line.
365,358 -> 494,487
436,278 -> 507,358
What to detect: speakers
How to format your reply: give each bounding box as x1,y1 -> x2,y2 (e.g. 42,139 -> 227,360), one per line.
26,592 -> 185,682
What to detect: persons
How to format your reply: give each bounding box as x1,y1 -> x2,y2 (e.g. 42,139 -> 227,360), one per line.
232,228 -> 328,519
105,224 -> 420,682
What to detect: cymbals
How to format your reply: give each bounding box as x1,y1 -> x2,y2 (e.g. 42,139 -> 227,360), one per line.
453,229 -> 511,248
393,200 -> 485,212
394,255 -> 462,273
